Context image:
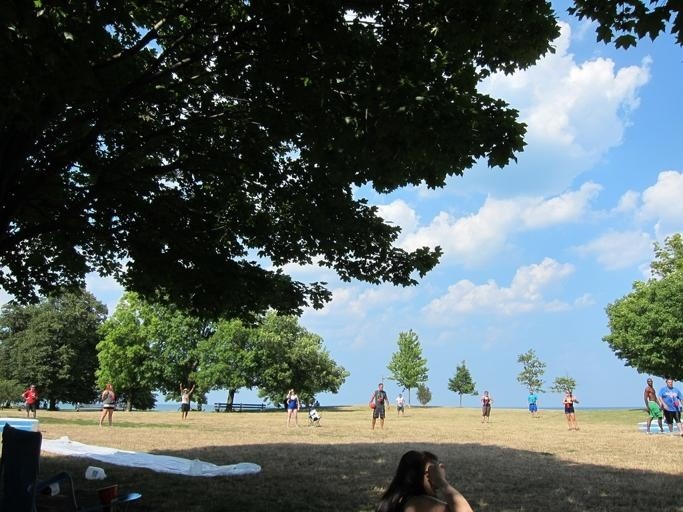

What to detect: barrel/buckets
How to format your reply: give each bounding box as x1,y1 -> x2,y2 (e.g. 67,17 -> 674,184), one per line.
85,465 -> 107,480
96,482 -> 118,504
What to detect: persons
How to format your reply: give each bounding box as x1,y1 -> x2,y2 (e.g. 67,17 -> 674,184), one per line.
314,400 -> 319,406
562,389 -> 579,431
43,401 -> 47,408
643,378 -> 665,434
527,390 -> 537,415
394,394 -> 405,415
286,389 -> 300,429
97,383 -> 116,430
20,383 -> 39,418
309,408 -> 319,424
376,449 -> 473,512
368,382 -> 390,429
480,390 -> 494,422
657,379 -> 682,435
179,382 -> 195,420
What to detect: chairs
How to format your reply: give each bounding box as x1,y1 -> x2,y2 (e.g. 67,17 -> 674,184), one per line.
0,422 -> 80,511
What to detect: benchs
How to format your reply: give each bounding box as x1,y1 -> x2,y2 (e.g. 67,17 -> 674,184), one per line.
213,402 -> 266,412
